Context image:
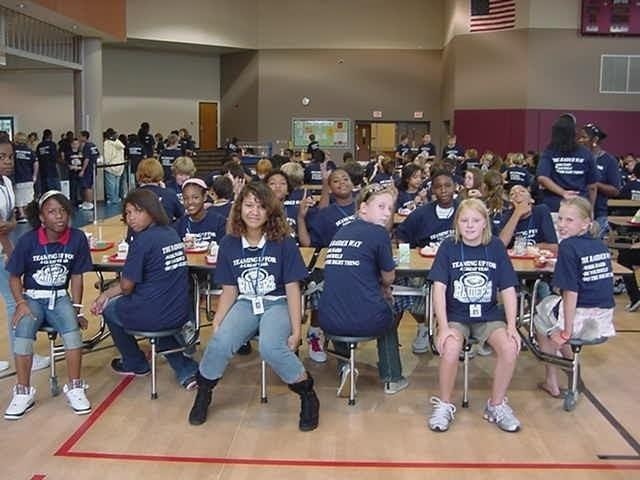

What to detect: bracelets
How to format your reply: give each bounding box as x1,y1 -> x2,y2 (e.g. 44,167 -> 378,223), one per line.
77,314 -> 85,318
71,303 -> 85,308
560,331 -> 570,341
14,300 -> 27,308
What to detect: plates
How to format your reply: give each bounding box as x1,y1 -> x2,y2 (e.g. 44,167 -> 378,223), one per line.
205,255 -> 217,265
418,247 -> 436,257
626,220 -> 640,225
507,248 -> 559,259
183,241 -> 209,254
107,252 -> 126,263
89,242 -> 114,252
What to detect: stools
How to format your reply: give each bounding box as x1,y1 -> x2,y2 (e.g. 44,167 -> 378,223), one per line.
42,310 -> 121,393
130,274 -> 202,398
425,290 -> 481,408
252,336 -> 275,403
509,277 -> 607,412
327,336 -> 374,408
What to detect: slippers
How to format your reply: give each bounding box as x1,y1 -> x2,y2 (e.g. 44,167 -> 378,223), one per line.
539,381 -> 563,399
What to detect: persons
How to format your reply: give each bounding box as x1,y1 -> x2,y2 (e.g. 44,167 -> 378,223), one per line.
93,189 -> 202,390
104,127 -> 125,204
534,196 -> 615,394
537,112 -> 596,218
126,134 -> 145,191
394,133 -> 538,169
614,152 -> 640,313
0,134 -> 51,374
272,135 -> 394,172
159,134 -> 183,173
138,122 -> 198,157
212,136 -> 271,182
429,197 -> 522,432
36,129 -> 66,193
135,157 -> 184,225
26,132 -> 40,149
296,167 -> 359,362
57,130 -> 75,159
170,178 -> 228,245
395,170 -> 464,354
577,121 -> 619,238
316,182 -> 408,397
456,168 -> 484,199
478,171 -> 514,217
171,154 -> 197,204
79,132 -> 100,211
394,165 -> 425,215
4,189 -> 91,420
263,170 -> 296,242
186,181 -> 320,431
65,139 -> 83,206
10,133 -> 38,224
497,182 -> 559,259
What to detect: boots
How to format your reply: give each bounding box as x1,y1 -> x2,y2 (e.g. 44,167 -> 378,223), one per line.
288,372 -> 319,431
189,370 -> 223,426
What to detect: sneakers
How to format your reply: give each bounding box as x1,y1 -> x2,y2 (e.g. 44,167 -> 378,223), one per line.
428,396 -> 457,432
63,379 -> 92,414
31,353 -> 50,371
412,325 -> 430,353
384,377 -> 408,394
111,358 -> 152,376
0,360 -> 9,372
307,327 -> 328,363
335,364 -> 360,397
625,299 -> 640,312
483,397 -> 520,432
4,384 -> 38,420
